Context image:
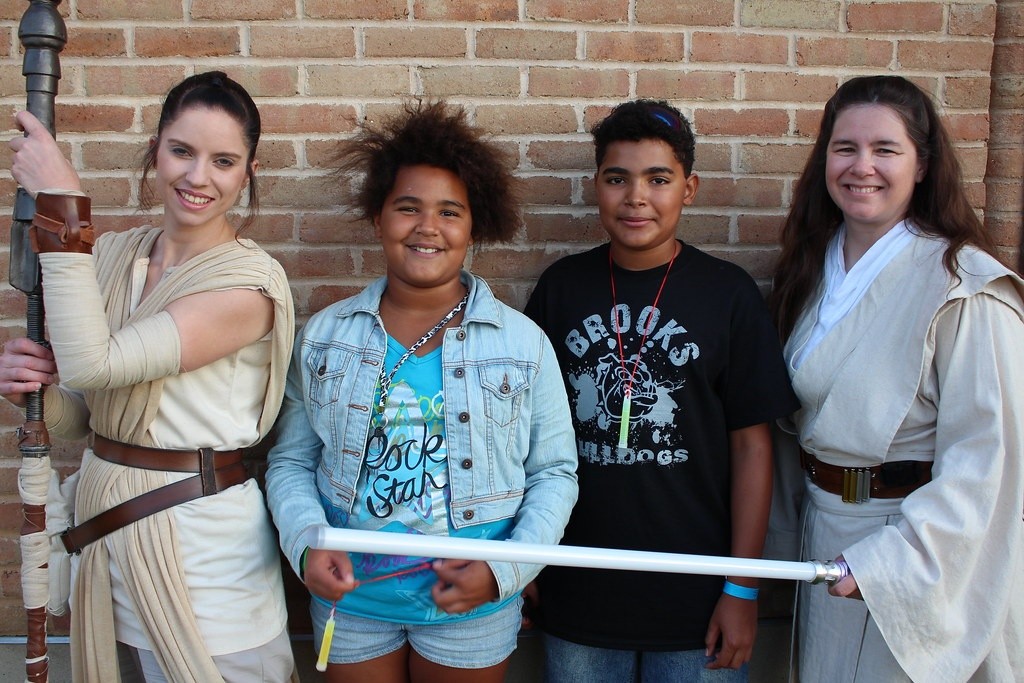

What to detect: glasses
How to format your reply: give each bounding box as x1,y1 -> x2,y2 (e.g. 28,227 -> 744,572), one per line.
605,102 -> 690,133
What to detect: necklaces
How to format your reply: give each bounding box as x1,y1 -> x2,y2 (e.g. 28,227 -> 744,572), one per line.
371,290 -> 468,436
316,563 -> 431,671
609,244 -> 676,448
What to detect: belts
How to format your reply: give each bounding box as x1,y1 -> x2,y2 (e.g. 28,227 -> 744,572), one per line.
59,430 -> 263,556
800,447 -> 934,506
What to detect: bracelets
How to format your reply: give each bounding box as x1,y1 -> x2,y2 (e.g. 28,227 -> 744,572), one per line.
723,581 -> 759,601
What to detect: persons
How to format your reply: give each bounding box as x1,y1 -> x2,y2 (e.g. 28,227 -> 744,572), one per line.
769,76 -> 1023,683
522,100 -> 801,682
0,72 -> 302,683
266,98 -> 579,682
